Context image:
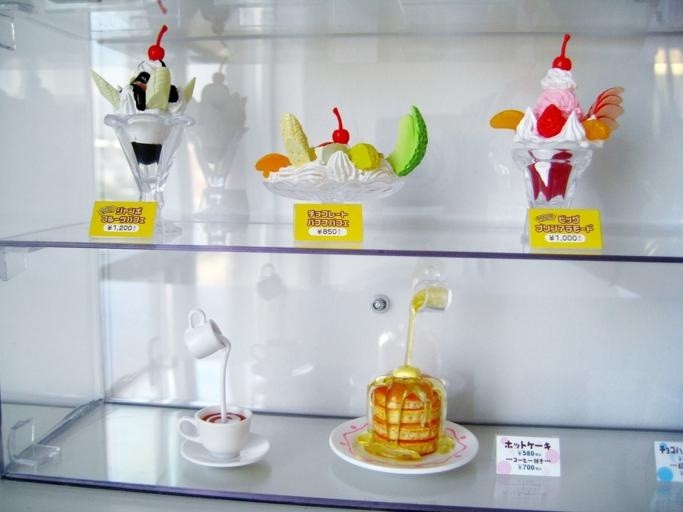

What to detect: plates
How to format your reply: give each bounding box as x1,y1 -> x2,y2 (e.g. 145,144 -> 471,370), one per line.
181,435 -> 272,468
329,415 -> 480,474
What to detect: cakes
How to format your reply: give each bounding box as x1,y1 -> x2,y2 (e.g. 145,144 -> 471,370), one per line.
355,286 -> 455,461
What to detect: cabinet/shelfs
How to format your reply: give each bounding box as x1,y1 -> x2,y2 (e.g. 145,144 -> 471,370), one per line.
0,2 -> 683,511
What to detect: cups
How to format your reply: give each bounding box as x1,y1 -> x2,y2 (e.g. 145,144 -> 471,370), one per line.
508,145 -> 594,233
179,305 -> 225,357
413,269 -> 451,315
177,405 -> 253,460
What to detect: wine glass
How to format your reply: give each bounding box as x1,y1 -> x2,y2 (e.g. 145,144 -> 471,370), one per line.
189,121 -> 246,227
109,117 -> 188,231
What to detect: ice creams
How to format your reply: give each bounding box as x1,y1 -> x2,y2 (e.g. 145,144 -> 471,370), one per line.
89,24 -> 195,164
489,33 -> 625,206
254,104 -> 428,203
